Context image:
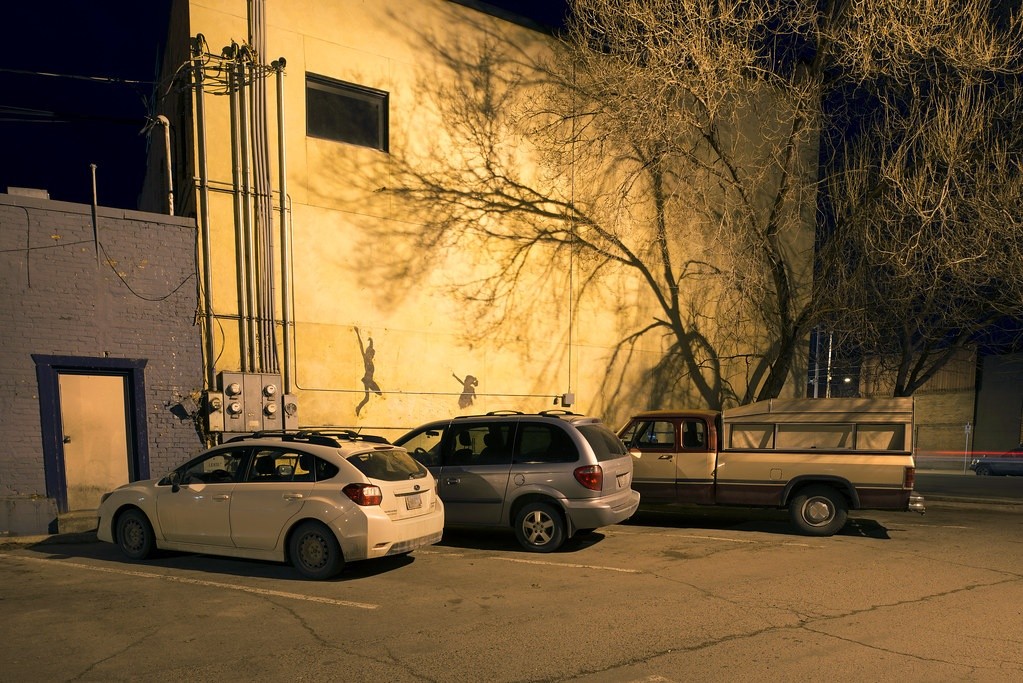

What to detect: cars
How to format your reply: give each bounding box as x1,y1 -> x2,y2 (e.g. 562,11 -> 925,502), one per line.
968,448 -> 1023,477
96,425 -> 445,581
365,408 -> 641,553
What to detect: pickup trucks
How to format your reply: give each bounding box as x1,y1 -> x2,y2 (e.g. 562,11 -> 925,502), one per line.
614,408 -> 927,537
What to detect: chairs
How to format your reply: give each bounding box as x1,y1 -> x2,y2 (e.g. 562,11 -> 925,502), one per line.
294,455 -> 320,481
450,431 -> 473,465
251,456 -> 277,482
476,434 -> 490,465
684,431 -> 698,447
